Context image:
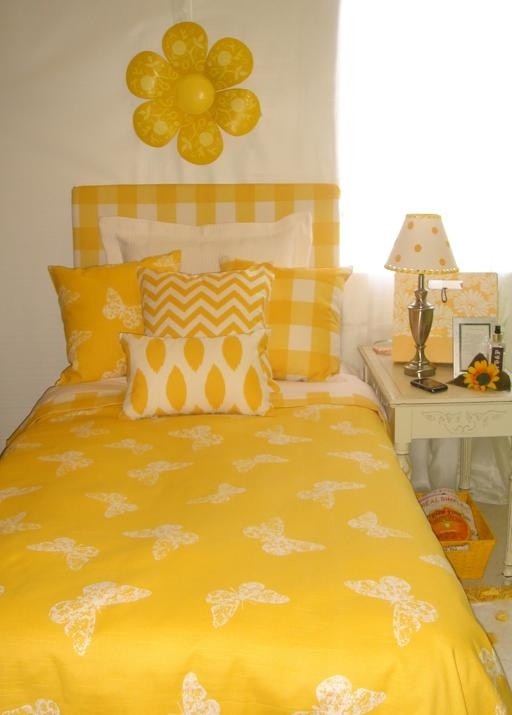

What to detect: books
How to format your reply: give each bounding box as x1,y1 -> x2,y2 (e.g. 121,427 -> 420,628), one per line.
419,488 -> 480,543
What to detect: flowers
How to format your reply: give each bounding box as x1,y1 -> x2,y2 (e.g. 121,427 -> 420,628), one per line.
449,353 -> 510,392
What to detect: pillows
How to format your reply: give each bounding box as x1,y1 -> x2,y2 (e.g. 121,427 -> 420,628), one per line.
220,257 -> 354,383
116,328 -> 274,419
138,260 -> 277,340
48,250 -> 184,385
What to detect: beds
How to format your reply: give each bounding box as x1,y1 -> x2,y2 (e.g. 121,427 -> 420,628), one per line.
3,184 -> 509,714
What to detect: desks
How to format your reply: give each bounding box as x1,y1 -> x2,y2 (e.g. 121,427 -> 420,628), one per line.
354,334 -> 511,587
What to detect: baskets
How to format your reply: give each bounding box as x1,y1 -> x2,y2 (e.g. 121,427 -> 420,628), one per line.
416,491 -> 496,580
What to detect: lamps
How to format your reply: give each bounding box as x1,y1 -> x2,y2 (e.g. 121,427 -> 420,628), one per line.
380,213 -> 459,378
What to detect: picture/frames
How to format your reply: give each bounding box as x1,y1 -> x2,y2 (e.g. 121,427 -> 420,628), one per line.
451,316 -> 498,381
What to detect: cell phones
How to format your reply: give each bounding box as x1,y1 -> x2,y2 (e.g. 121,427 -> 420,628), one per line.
411,378 -> 448,393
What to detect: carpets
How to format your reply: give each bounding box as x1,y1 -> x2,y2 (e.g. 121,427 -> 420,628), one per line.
463,580 -> 511,691
